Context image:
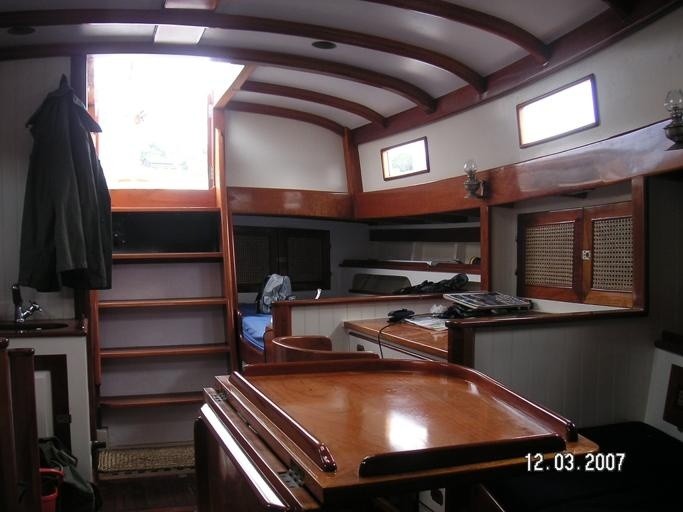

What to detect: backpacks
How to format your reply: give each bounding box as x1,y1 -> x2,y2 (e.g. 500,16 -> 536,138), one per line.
255,272 -> 296,315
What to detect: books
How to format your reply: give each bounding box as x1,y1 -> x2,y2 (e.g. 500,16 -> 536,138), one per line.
442,289 -> 529,309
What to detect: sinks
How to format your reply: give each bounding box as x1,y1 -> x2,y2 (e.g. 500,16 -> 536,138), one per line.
0,320 -> 73,331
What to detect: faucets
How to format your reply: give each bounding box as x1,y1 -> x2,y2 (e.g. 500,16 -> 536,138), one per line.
11,284 -> 43,321
